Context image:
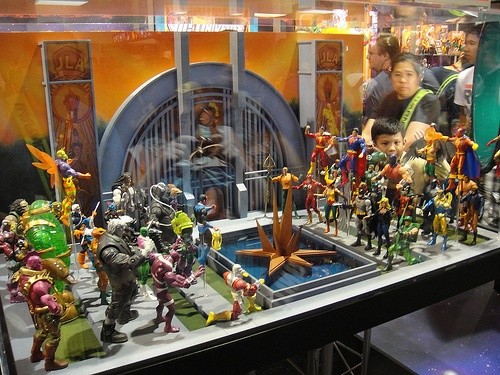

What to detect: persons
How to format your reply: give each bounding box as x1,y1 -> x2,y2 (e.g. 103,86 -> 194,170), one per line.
429,54 -> 474,127
266,125 -> 485,271
363,32 -> 439,115
127,80 -> 274,222
370,118 -> 428,201
487,134 -> 500,177
377,52 -> 438,126
453,23 -> 485,140
205,263 -> 266,326
0,144 -> 222,372
